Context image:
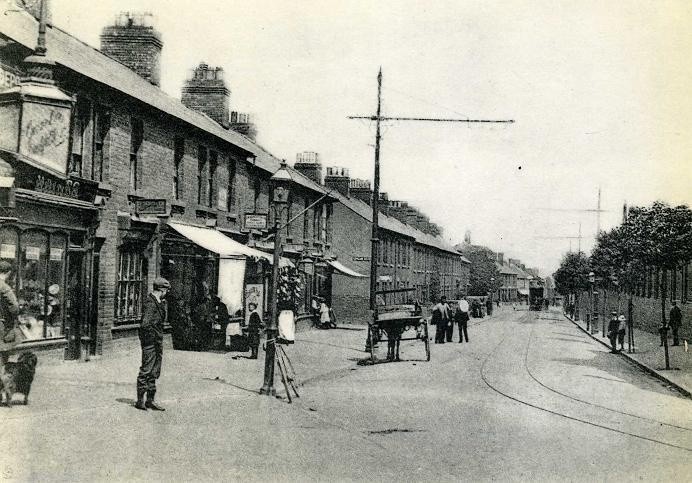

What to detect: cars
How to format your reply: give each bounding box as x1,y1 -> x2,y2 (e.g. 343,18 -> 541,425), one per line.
555,296 -> 565,305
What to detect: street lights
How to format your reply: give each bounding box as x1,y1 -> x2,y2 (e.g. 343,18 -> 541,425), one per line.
259,153 -> 292,394
489,276 -> 495,317
585,269 -> 596,335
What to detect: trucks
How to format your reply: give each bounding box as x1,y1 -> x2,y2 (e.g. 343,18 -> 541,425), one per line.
528,285 -> 544,310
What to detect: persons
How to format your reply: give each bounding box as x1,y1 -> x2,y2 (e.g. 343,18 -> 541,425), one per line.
430,295 -> 454,345
455,294 -> 470,343
131,277 -> 172,413
246,302 -> 262,361
667,302 -> 684,346
606,311 -> 620,354
0,260 -> 21,402
657,318 -> 670,347
616,315 -> 627,350
212,295 -> 230,350
486,297 -> 492,315
445,302 -> 456,344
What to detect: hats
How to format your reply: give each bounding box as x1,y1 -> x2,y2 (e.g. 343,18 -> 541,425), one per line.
152,277 -> 172,290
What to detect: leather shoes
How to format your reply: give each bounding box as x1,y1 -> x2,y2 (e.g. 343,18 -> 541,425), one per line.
145,401 -> 165,411
135,400 -> 147,410
